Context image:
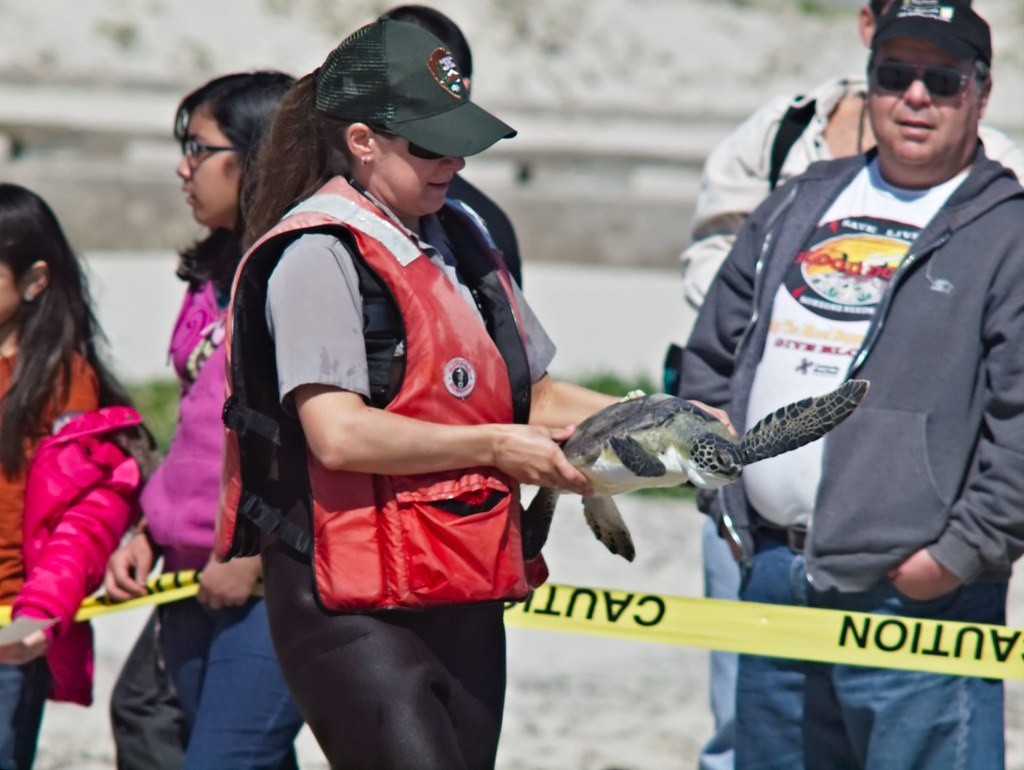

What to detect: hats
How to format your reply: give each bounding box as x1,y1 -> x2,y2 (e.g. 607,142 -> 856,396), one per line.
871,0 -> 992,67
314,19 -> 519,159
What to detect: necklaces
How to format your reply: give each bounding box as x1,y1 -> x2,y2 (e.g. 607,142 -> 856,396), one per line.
857,98 -> 868,154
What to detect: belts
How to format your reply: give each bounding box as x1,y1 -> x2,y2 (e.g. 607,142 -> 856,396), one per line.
758,524 -> 808,553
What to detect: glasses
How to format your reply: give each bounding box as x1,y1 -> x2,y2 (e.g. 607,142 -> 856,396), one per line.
181,141 -> 249,159
367,123 -> 446,161
873,56 -> 978,98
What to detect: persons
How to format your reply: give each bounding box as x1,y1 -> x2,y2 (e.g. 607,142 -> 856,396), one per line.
682,0 -> 1023,770
185,3 -> 523,770
226,20 -> 739,770
0,181 -> 156,770
105,70 -> 298,770
